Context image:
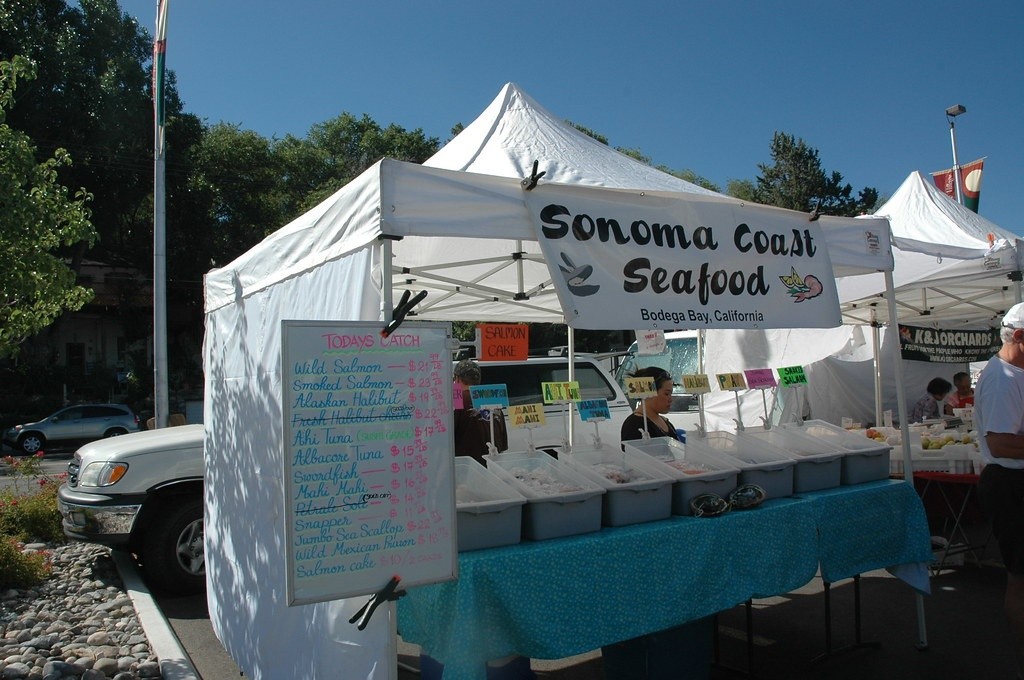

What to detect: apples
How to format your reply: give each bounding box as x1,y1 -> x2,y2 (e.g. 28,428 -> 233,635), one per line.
866,427 -> 884,439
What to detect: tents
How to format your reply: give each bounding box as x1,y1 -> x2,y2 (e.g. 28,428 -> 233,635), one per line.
705,170 -> 1024,428
202,83 -> 926,680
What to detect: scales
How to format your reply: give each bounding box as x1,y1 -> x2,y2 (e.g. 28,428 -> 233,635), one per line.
921,416 -> 966,428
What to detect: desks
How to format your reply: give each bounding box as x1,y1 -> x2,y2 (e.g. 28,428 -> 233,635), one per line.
396,471 -> 994,680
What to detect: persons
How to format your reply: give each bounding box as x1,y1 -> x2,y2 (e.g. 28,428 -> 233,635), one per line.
910,377 -> 952,423
620,366 -> 681,456
453,360 -> 508,469
945,372 -> 976,416
975,301 -> 1024,608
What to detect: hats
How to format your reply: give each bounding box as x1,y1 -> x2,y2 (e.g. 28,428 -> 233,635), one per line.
1000,302 -> 1024,330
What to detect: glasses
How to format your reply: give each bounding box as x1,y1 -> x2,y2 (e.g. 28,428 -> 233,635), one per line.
655,371 -> 672,381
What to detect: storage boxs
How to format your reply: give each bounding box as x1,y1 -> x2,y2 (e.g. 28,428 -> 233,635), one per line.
455,407 -> 983,554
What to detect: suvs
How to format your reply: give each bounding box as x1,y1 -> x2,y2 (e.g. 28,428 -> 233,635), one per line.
2,403 -> 141,455
57,349 -> 635,594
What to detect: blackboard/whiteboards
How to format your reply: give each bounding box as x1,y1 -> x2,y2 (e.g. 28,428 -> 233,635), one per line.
277,320 -> 460,608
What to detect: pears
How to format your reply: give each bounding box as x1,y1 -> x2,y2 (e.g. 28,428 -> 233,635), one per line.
921,435 -> 972,449
